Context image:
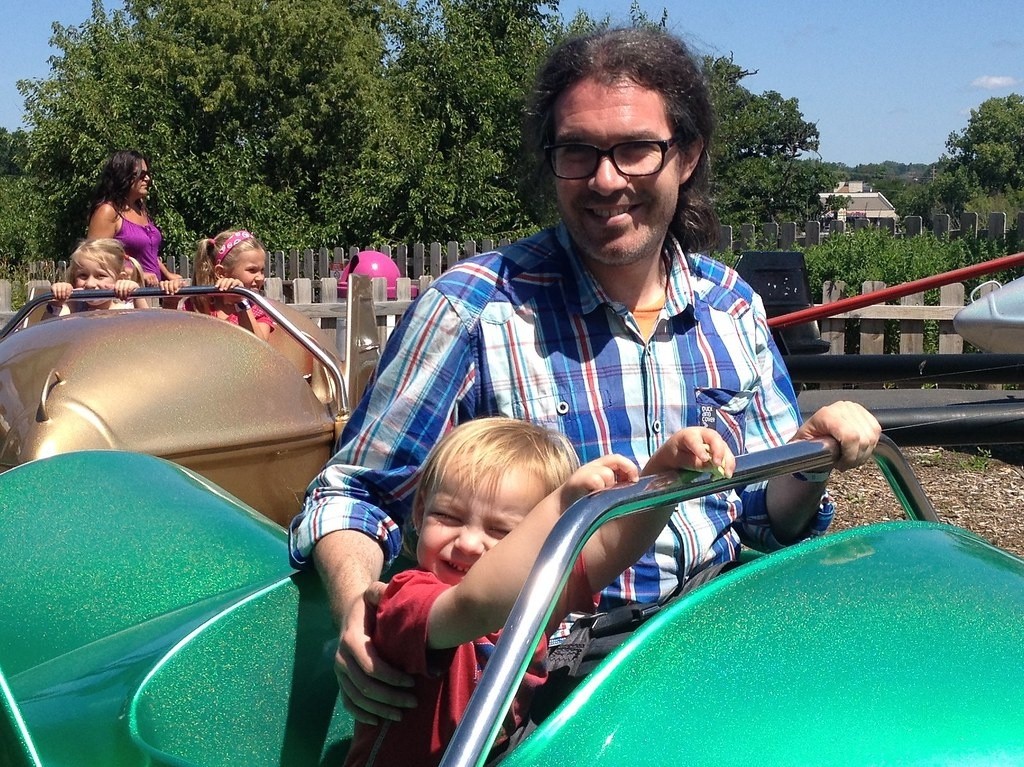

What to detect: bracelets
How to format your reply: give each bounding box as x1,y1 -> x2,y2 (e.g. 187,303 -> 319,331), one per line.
234,298 -> 251,313
45,304 -> 62,316
790,467 -> 834,483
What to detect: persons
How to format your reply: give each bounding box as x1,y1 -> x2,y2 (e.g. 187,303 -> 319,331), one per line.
287,23 -> 881,767
343,416 -> 736,767
86,150 -> 187,288
40,238 -> 149,322
160,230 -> 275,345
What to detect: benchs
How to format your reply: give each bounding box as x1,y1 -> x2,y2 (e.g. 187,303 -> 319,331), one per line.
24,286 -> 340,407
0,450 -> 307,767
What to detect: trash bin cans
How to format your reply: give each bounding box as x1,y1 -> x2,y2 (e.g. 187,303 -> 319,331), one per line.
337,251 -> 418,303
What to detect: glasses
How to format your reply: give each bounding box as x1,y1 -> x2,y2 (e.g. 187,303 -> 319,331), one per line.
132,170 -> 150,177
540,134 -> 683,181
